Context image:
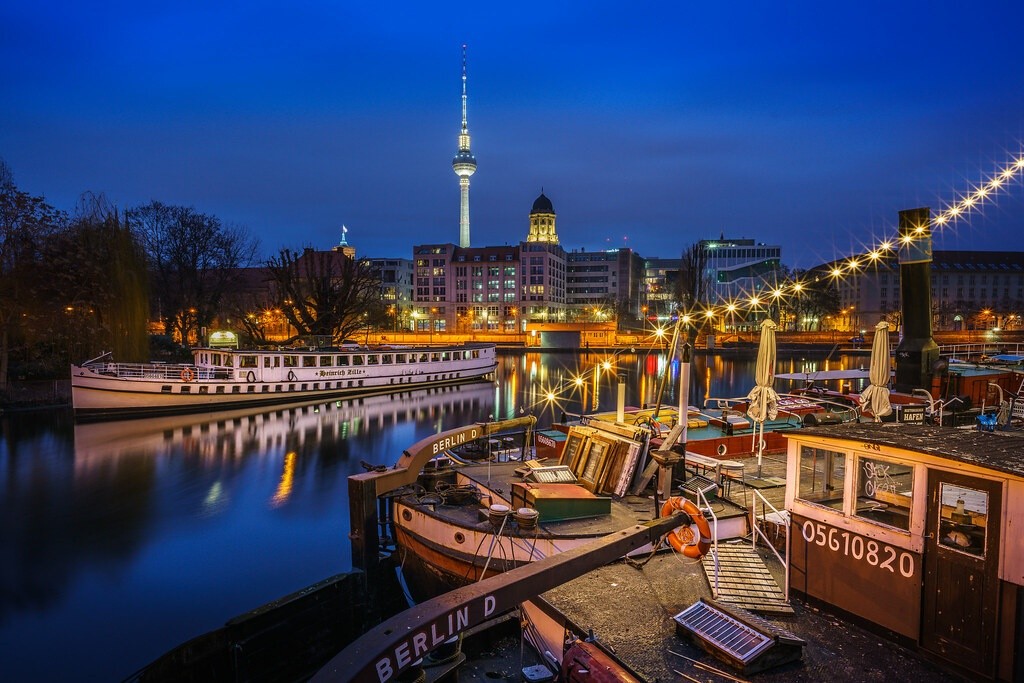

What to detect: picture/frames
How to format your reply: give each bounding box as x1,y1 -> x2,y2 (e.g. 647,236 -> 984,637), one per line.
577,437 -> 611,493
558,431 -> 587,475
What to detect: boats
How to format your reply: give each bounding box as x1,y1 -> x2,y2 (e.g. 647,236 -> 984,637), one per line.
121,207 -> 1024,683
71,346 -> 499,426
74,380 -> 502,470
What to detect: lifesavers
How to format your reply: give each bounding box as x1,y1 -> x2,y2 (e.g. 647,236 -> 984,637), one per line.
181,369 -> 194,381
247,371 -> 255,383
660,496 -> 711,559
288,371 -> 294,381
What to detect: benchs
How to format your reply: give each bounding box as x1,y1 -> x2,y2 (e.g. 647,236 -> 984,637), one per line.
686,460 -> 743,496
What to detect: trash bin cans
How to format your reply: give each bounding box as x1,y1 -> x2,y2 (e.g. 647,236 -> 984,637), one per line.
706,334 -> 715,349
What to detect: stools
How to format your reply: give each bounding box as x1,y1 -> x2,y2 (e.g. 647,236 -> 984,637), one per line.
716,459 -> 747,507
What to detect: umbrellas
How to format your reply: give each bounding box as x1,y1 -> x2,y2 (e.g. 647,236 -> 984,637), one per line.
746,319 -> 781,478
859,321 -> 892,422
